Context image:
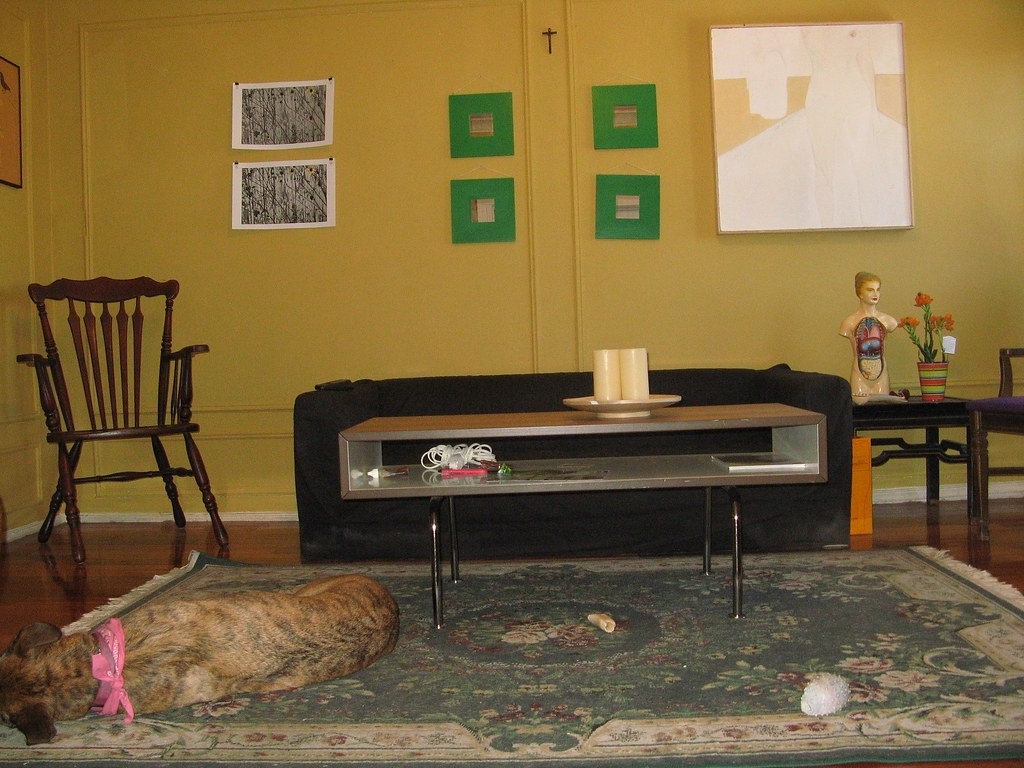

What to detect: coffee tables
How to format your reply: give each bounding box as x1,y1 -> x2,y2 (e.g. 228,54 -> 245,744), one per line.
338,404 -> 828,629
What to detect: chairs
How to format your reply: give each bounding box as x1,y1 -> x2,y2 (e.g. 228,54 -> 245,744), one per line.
975,348 -> 1024,520
14,275 -> 231,564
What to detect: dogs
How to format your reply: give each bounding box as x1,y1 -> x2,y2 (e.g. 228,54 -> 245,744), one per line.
0,573 -> 400,747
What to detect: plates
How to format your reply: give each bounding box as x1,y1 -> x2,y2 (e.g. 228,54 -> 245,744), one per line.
562,394 -> 683,419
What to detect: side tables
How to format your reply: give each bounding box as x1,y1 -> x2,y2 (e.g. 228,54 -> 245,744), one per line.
853,395 -> 981,519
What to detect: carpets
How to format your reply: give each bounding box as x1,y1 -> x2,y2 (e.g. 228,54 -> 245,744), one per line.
0,547 -> 1024,768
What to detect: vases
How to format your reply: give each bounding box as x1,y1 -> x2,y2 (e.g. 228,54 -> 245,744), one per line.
916,361 -> 949,402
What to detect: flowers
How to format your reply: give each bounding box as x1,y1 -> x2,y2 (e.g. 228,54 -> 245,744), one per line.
898,292 -> 955,363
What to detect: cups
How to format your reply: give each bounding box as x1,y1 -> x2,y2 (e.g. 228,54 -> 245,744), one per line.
592,349 -> 622,403
621,348 -> 649,401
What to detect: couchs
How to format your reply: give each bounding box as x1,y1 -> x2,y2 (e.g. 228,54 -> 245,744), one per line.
291,363 -> 852,563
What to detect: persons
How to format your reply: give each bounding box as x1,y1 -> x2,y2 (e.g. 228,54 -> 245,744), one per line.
838,271 -> 898,395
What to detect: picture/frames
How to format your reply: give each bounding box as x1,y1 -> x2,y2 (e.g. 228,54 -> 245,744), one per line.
0,56 -> 23,189
707,19 -> 915,236
